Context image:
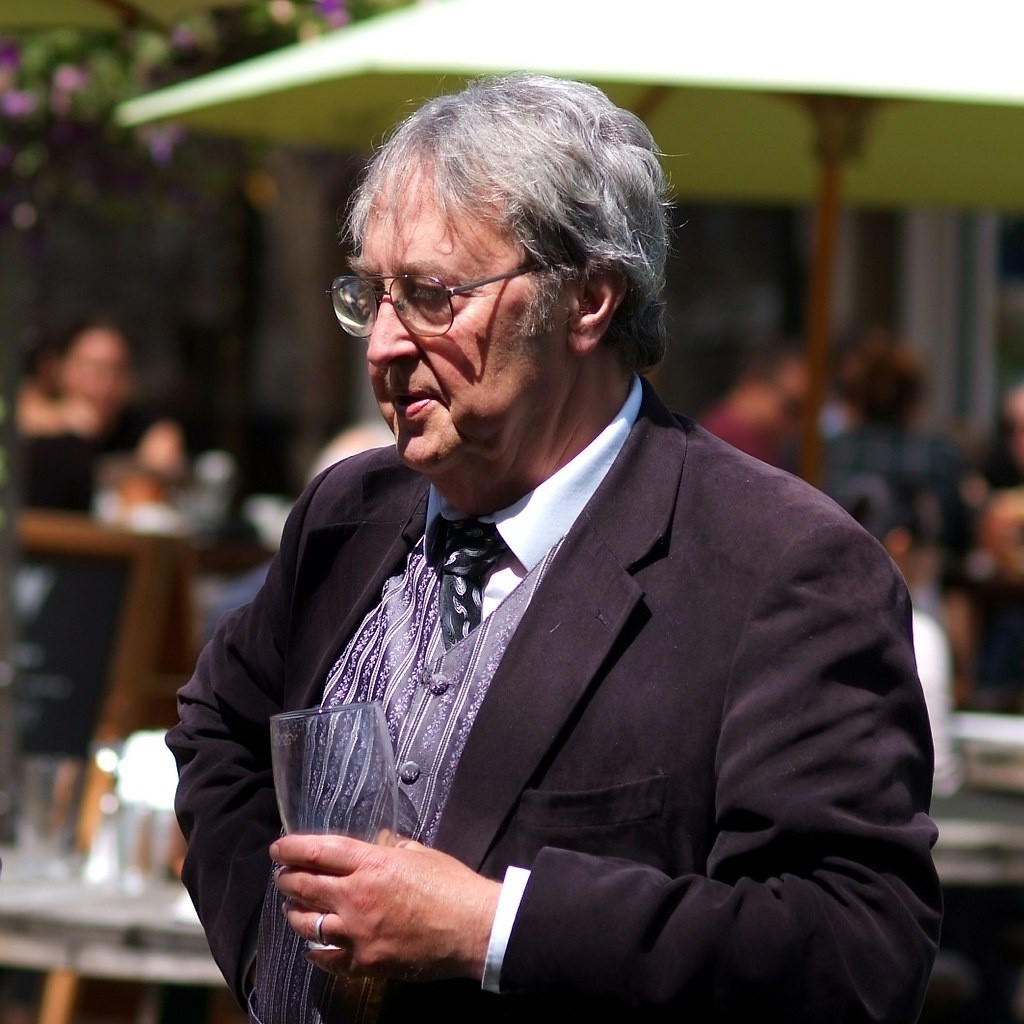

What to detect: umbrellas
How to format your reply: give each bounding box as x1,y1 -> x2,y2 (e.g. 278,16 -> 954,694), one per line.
107,1 -> 1024,492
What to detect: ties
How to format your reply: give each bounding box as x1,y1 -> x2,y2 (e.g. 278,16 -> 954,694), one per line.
436,520 -> 500,652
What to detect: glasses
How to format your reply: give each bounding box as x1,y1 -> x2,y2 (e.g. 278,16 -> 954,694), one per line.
324,261 -> 548,340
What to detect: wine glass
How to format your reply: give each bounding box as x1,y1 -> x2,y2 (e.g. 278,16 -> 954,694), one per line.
271,702 -> 398,952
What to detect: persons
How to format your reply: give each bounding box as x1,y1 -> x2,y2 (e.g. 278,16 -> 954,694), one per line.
163,69 -> 949,1024
891,381 -> 1024,791
11,312 -> 406,767
692,340 -> 828,476
813,332 -> 945,572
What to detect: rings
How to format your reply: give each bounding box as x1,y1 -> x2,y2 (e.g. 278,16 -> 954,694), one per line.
314,912 -> 333,948
